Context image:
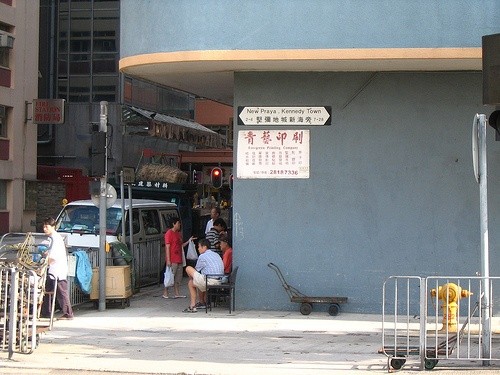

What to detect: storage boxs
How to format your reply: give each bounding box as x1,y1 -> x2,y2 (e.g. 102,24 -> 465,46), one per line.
90,265 -> 132,300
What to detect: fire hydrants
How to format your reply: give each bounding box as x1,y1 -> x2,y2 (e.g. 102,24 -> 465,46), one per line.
429,283 -> 473,332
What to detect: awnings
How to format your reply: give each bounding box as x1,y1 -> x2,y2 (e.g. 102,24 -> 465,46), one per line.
130,107 -> 217,134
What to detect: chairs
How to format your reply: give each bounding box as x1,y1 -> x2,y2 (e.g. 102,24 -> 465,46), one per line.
206,266 -> 238,314
73,210 -> 94,229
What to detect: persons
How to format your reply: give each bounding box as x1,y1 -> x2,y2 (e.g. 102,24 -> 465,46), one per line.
205,207 -> 221,235
162,217 -> 197,299
205,218 -> 227,302
195,236 -> 233,309
182,239 -> 224,313
43,216 -> 75,320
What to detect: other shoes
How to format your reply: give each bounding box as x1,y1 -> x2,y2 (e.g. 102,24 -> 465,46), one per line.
57,314 -> 73,320
182,306 -> 197,312
196,302 -> 206,309
175,295 -> 186,298
162,294 -> 170,298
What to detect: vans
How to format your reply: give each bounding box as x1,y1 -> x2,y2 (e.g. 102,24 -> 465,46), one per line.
50,198 -> 183,278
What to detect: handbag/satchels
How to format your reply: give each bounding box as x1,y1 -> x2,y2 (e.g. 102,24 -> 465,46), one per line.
187,238 -> 198,260
164,265 -> 174,286
181,246 -> 186,266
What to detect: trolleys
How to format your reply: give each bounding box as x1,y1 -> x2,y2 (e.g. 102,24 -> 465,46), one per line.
267,262 -> 348,316
93,256 -> 135,309
378,292 -> 485,370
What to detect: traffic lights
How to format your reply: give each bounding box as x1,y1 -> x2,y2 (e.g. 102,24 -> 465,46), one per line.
488,109 -> 500,141
229,174 -> 234,190
210,167 -> 222,190
194,173 -> 198,184
